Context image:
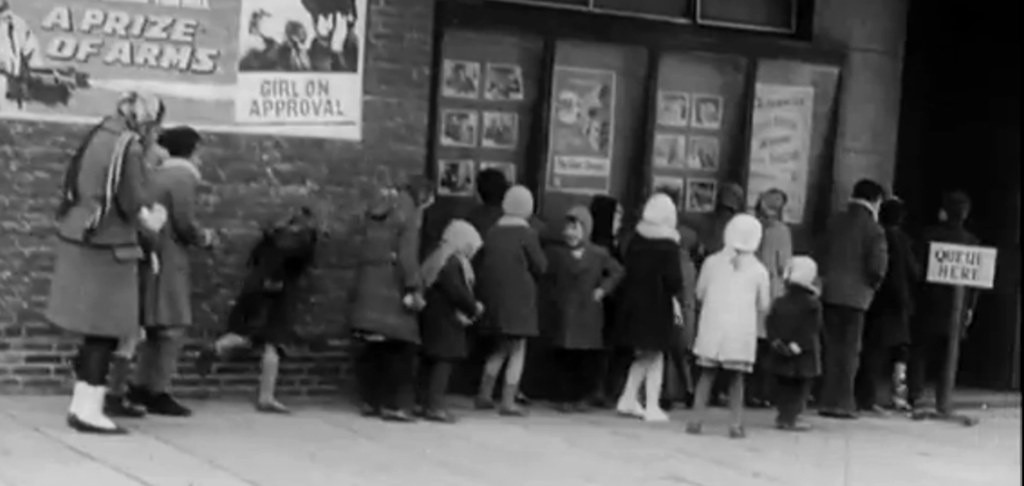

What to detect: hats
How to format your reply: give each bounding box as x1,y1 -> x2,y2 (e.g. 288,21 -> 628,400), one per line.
644,193 -> 676,228
723,214 -> 762,251
718,183 -> 743,209
502,186 -> 532,218
784,256 -> 819,293
563,204 -> 591,242
441,219 -> 483,259
760,189 -> 786,215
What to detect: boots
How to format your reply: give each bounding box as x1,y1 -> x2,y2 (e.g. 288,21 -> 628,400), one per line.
196,334 -> 250,378
500,380 -> 528,415
475,373 -> 496,409
891,361 -> 912,412
68,380 -> 128,435
257,354 -> 290,414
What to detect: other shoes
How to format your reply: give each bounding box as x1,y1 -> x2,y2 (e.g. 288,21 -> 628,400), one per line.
642,411 -> 670,423
686,421 -> 701,434
618,397 -> 645,417
912,400 -> 937,418
379,407 -> 414,422
414,404 -> 453,421
104,394 -> 143,417
149,391 -> 191,416
361,403 -> 378,415
730,425 -> 744,438
126,380 -> 149,404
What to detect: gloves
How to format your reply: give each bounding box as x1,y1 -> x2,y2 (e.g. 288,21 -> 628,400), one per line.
774,339 -> 801,357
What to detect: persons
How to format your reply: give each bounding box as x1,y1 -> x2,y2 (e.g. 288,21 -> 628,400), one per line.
348,163 -> 981,439
193,207 -> 319,415
103,123 -> 218,414
41,88 -> 166,433
